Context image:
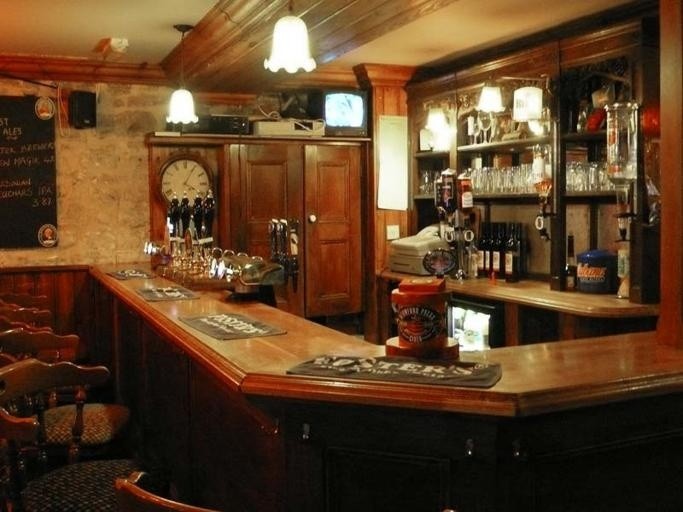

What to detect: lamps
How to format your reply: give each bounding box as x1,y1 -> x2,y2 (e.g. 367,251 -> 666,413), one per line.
476,73 -> 556,114
513,87 -> 548,120
264,1 -> 316,73
166,25 -> 199,124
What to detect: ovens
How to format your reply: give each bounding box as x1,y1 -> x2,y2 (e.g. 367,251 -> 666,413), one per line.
446,298 -> 502,356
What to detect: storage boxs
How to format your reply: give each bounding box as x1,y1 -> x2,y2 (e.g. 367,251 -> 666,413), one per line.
84,325 -> 113,363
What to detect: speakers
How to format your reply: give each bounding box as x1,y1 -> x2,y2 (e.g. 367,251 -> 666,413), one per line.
69,90 -> 96,129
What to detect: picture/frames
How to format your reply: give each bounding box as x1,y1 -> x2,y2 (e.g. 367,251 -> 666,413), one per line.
35,96 -> 55,120
38,224 -> 58,247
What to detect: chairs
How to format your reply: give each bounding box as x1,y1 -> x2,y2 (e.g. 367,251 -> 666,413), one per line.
0,292 -> 47,311
0,311 -> 96,368
114,471 -> 221,512
0,359 -> 176,511
0,328 -> 91,401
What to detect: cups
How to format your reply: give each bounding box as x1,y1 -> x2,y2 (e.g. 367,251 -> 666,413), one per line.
467,159 -> 613,193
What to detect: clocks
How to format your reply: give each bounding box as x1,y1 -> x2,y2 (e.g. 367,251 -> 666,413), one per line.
157,155 -> 215,215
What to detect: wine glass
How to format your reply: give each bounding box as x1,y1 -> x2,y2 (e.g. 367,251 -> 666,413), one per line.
469,111 -> 492,145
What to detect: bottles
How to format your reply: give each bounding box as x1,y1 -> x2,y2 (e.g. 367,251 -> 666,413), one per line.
473,219 -> 529,283
561,234 -> 577,291
422,170 -> 431,195
168,240 -> 213,276
603,102 -> 640,240
531,143 -> 553,211
432,168 -> 473,229
167,188 -> 214,239
574,104 -> 606,133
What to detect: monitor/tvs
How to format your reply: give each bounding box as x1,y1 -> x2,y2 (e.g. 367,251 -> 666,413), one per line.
320,87 -> 369,137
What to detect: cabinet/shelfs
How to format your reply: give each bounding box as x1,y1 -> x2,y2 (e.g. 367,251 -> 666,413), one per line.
407,33 -> 659,345
369,85 -> 407,344
145,137 -> 372,318
